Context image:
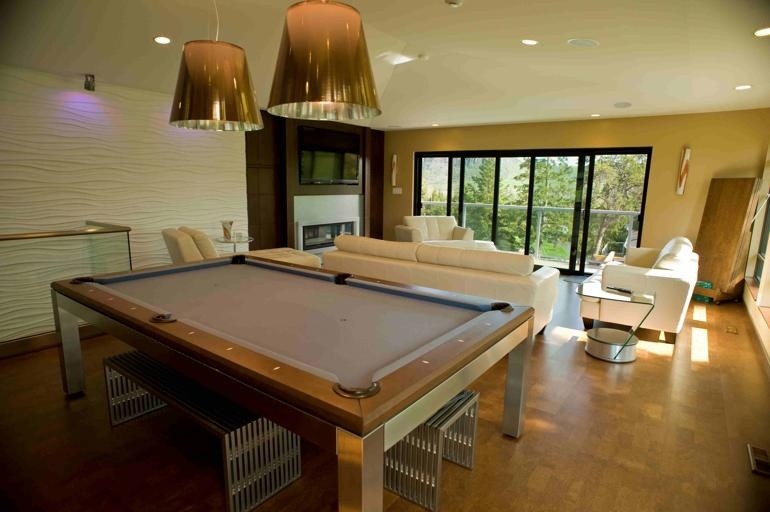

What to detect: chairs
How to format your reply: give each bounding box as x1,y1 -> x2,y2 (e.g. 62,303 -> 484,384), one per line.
579,236 -> 700,334
394,215 -> 475,242
161,228 -> 322,270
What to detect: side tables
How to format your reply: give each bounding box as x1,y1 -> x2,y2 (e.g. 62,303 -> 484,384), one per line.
575,282 -> 656,364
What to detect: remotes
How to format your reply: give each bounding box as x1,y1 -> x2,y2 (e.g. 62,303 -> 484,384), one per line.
606,285 -> 634,294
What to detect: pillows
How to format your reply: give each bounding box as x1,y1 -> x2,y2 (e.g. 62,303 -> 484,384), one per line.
177,226 -> 220,259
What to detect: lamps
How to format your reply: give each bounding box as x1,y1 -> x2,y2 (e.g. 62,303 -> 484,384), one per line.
266,0 -> 382,123
675,145 -> 690,198
390,155 -> 397,188
169,0 -> 265,133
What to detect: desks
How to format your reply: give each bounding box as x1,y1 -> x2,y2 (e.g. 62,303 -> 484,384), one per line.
50,254 -> 535,512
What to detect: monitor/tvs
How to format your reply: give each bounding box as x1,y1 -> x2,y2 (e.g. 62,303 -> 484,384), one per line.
297,125 -> 361,185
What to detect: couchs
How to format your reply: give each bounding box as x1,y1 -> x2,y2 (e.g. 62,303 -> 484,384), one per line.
322,234 -> 560,337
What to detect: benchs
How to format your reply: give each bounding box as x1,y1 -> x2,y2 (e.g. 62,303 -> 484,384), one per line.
102,350 -> 303,512
383,389 -> 482,512
